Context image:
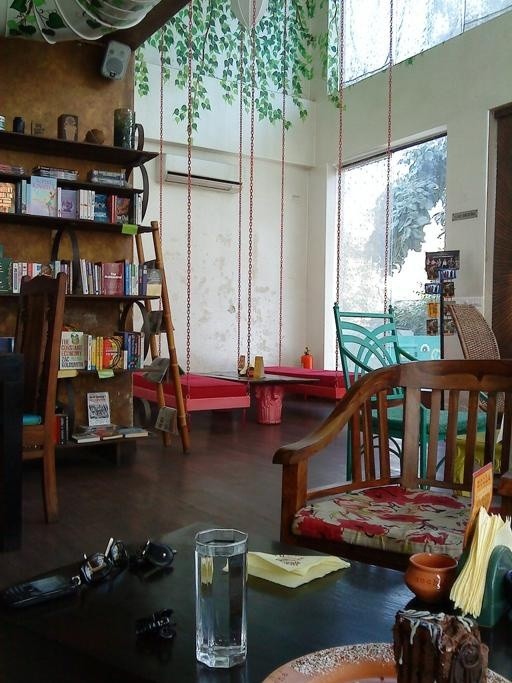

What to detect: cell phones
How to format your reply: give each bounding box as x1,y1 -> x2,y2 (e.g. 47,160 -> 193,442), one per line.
3,574 -> 75,608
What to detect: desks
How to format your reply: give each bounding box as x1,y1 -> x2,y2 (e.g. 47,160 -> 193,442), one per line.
453,434 -> 504,484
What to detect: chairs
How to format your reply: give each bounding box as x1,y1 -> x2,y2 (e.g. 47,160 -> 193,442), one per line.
450,303 -> 508,416
333,303 -> 490,490
13,272 -> 69,524
273,358 -> 507,562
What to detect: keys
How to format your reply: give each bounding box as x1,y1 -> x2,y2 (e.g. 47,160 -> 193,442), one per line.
130,535 -> 177,582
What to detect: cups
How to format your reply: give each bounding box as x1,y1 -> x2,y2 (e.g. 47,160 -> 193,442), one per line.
403,553 -> 459,598
193,527 -> 250,671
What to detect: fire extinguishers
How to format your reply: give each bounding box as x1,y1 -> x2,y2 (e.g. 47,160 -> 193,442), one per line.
301,346 -> 314,369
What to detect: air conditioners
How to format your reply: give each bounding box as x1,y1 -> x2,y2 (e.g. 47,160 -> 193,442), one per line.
155,152 -> 247,194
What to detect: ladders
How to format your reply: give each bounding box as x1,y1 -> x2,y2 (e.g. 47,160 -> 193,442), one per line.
138,220 -> 192,456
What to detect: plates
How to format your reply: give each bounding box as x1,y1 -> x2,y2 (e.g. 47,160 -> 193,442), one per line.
263,643 -> 511,682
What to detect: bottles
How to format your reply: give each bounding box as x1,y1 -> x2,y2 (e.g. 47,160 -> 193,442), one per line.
254,356 -> 264,379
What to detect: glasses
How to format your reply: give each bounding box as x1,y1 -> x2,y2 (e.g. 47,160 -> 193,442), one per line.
72,537 -> 129,586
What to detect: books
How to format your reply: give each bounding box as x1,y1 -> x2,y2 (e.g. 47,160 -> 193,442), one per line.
0,165 -> 142,225
60,331 -> 145,369
1,257 -> 147,296
54,392 -> 148,443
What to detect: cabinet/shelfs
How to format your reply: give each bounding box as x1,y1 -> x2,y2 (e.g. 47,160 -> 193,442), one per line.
0,128 -> 158,466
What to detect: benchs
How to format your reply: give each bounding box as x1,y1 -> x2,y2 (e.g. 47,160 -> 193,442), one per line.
131,372 -> 252,433
265,366 -> 377,401
